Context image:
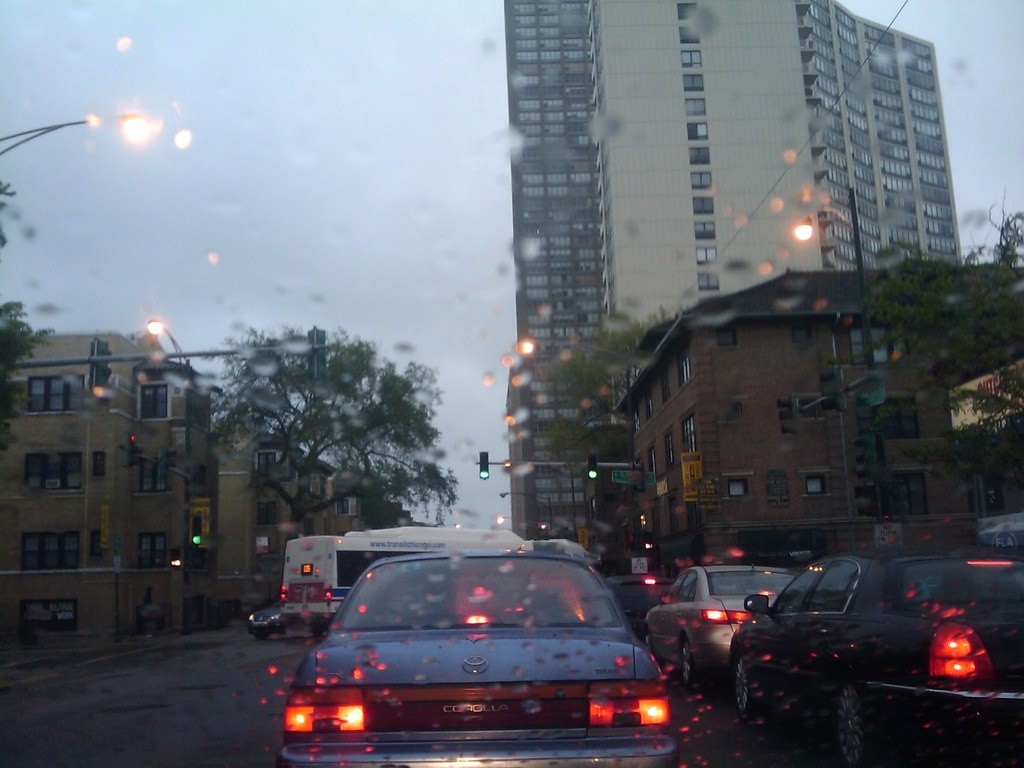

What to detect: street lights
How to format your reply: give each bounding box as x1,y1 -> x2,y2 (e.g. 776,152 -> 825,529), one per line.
146,317 -> 192,638
793,186 -> 891,521
499,491 -> 552,529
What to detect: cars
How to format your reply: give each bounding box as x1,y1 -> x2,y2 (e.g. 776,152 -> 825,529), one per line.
642,563 -> 801,683
728,545 -> 1024,767
273,550 -> 680,768
249,599 -> 284,642
603,574 -> 678,642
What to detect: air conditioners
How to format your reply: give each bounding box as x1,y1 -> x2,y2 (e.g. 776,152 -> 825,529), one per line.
49,603 -> 60,610
45,480 -> 60,488
173,386 -> 182,395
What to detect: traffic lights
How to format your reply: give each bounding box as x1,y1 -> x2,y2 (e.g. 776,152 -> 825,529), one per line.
587,454 -> 598,479
479,451 -> 489,480
126,429 -> 141,467
191,515 -> 203,545
170,547 -> 185,568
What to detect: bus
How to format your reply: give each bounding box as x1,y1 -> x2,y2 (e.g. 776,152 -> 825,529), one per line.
279,525 -> 600,636
